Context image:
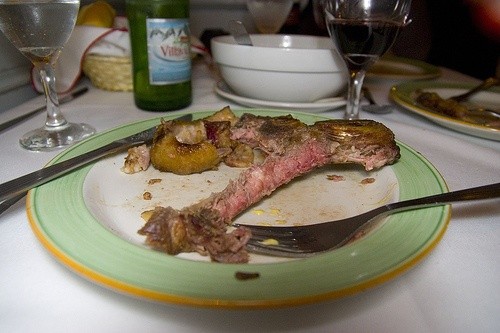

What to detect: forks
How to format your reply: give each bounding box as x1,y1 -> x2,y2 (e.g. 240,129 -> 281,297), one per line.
224,182 -> 500,258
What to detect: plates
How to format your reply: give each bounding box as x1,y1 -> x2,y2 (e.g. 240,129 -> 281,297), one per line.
24,110 -> 452,310
389,79 -> 500,142
363,55 -> 441,79
214,77 -> 346,114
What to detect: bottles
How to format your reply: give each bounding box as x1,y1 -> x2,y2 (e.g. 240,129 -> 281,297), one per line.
123,0 -> 193,113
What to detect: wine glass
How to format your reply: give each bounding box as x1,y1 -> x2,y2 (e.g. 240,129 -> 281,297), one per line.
322,0 -> 410,121
0,0 -> 96,154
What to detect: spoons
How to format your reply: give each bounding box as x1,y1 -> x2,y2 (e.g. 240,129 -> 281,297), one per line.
361,86 -> 393,114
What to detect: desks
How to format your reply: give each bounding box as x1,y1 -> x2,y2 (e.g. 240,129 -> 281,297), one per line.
0,68 -> 500,333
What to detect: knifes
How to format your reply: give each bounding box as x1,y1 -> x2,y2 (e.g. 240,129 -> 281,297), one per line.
0,113 -> 193,204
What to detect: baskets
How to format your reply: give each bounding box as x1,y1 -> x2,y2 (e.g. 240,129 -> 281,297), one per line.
80,53 -> 135,91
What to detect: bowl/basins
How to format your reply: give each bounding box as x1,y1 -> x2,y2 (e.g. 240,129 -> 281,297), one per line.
211,32 -> 347,103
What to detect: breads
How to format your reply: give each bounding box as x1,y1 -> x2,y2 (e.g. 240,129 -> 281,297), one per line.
75,1 -> 116,28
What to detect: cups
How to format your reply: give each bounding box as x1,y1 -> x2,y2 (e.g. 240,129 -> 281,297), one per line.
245,0 -> 293,34
